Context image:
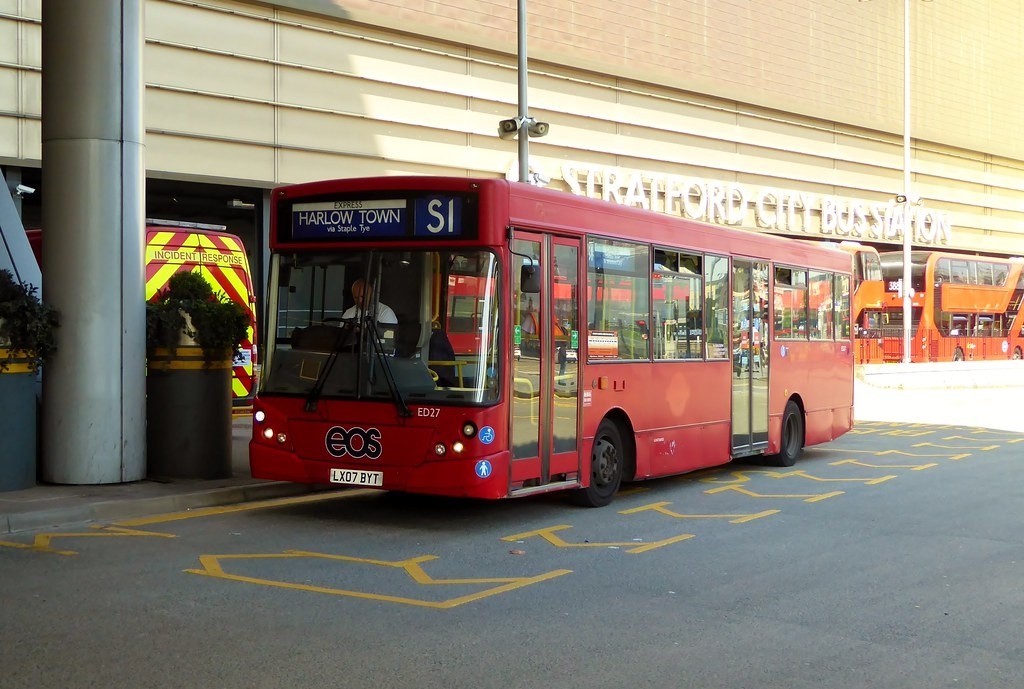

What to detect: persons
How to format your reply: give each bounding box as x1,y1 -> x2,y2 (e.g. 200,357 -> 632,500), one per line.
340,280 -> 397,357
559,344 -> 566,375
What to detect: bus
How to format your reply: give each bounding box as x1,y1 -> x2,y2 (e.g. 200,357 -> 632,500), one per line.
797,240 -> 1024,365
250,176 -> 855,507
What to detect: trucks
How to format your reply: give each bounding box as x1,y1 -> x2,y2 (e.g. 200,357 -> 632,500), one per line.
24,219 -> 258,422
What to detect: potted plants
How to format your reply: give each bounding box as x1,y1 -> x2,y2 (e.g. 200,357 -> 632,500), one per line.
145,269 -> 250,484
0,268 -> 66,492
